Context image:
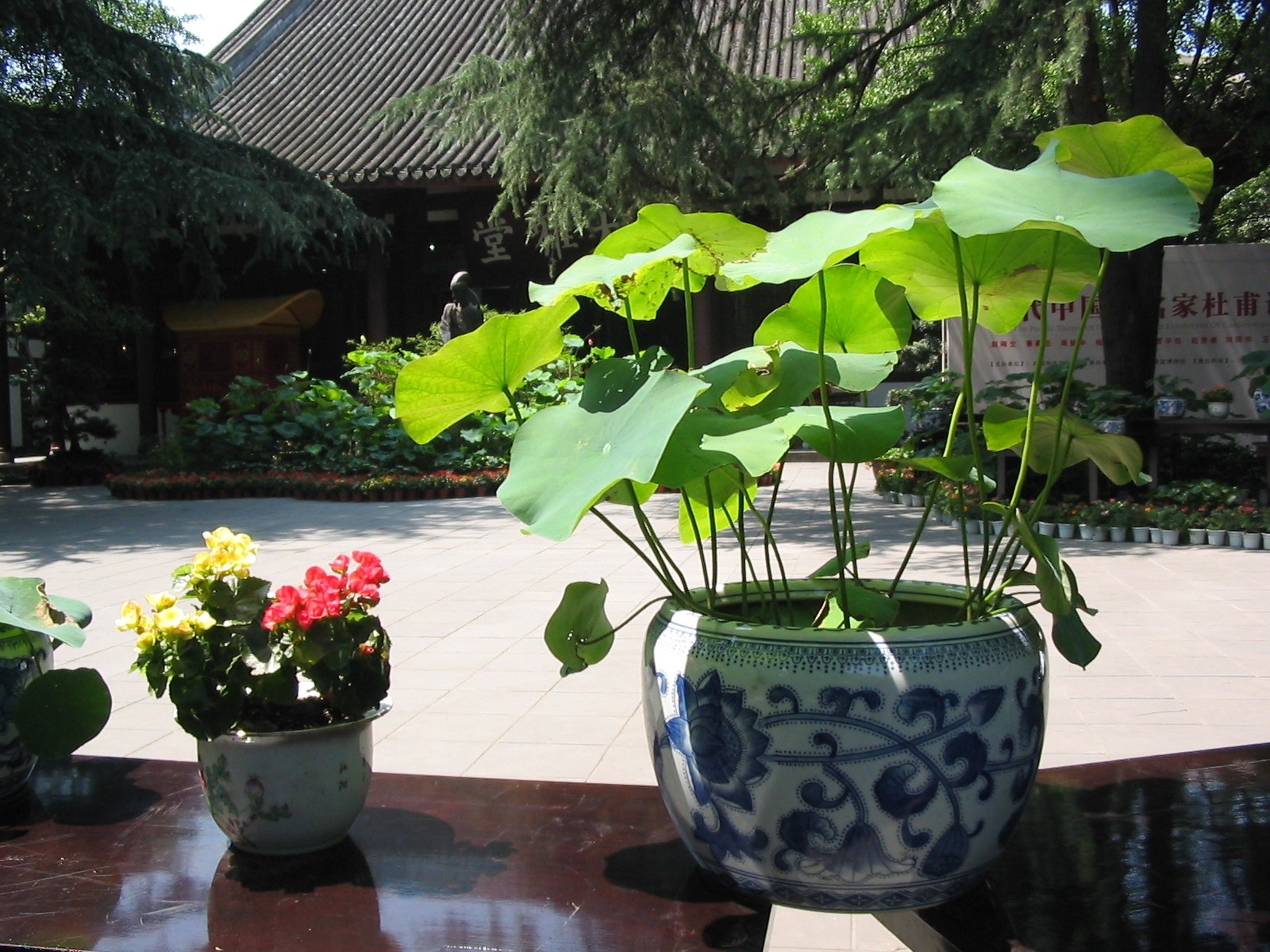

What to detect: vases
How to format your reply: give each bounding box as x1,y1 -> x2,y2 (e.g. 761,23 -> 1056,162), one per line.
1207,401 -> 1229,419
199,701 -> 391,855
110,484 -> 488,501
881,490 -> 1270,551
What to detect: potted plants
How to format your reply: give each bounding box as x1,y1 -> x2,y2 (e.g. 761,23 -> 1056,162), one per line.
1142,374 -> 1201,421
391,114 -> 1216,912
1085,384 -> 1141,435
1241,349 -> 1270,418
0,576 -> 112,801
887,373 -> 963,430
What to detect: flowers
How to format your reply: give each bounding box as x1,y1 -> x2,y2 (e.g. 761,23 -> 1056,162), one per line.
1203,383 -> 1234,403
106,469 -> 508,488
118,528 -> 394,739
876,467 -> 1270,533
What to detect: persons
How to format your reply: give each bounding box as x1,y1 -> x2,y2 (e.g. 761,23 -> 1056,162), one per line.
439,271 -> 483,344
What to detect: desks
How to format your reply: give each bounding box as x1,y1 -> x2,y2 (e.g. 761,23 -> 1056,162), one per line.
0,755 -> 773,952
870,742 -> 1270,952
